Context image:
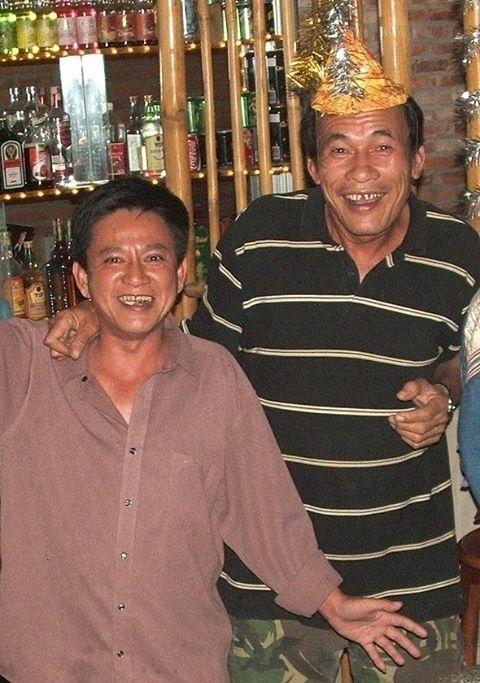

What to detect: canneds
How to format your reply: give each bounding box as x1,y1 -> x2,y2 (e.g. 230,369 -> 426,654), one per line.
242,129 -> 258,170
187,136 -> 202,170
244,50 -> 256,93
201,135 -> 208,169
269,106 -> 293,166
188,96 -> 200,135
200,96 -> 206,135
241,93 -> 257,129
265,49 -> 285,108
216,129 -> 235,171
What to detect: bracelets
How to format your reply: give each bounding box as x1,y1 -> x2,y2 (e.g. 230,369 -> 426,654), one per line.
432,381 -> 457,418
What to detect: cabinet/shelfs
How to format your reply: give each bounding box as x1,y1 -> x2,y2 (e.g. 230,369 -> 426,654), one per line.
0,0 -> 306,331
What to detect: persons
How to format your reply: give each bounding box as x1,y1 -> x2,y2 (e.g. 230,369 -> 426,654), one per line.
2,177 -> 430,683
41,95 -> 479,683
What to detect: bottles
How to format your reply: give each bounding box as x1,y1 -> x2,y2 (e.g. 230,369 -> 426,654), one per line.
47,217 -> 66,316
122,94 -> 143,173
60,220 -> 87,308
23,240 -> 47,321
104,102 -> 128,176
0,116 -> 26,191
50,94 -> 71,182
141,95 -> 164,176
23,87 -> 51,186
1,228 -> 25,317
6,86 -> 28,185
0,0 -> 158,51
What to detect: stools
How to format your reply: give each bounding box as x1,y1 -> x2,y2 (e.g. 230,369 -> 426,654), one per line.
458,527 -> 479,671
